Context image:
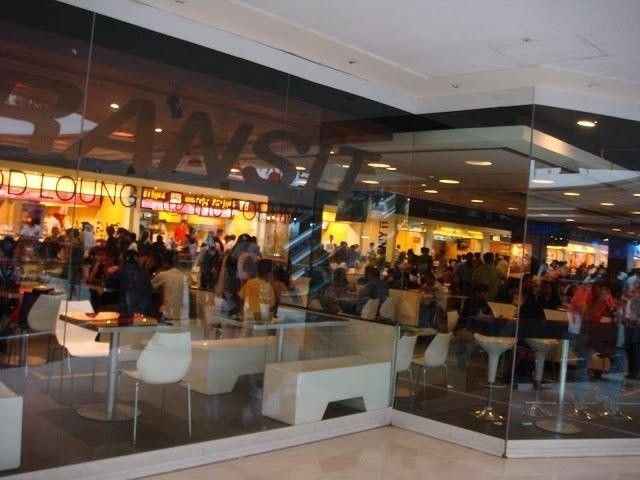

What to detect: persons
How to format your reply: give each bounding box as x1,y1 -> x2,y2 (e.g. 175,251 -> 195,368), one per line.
1,218 -> 639,374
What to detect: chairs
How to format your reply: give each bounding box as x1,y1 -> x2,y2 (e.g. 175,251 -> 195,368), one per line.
359,296 -> 380,321
47,299 -> 119,403
114,331 -> 193,448
446,311 -> 459,330
410,331 -> 451,403
7,292 -> 68,364
398,333 -> 416,411
379,298 -> 398,320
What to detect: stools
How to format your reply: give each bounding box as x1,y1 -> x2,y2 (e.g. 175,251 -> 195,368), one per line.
470,333 -> 517,421
522,334 -> 560,417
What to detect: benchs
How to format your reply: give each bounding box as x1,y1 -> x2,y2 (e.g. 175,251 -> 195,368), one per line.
0,379 -> 23,470
185,335 -> 299,397
261,355 -> 390,426
